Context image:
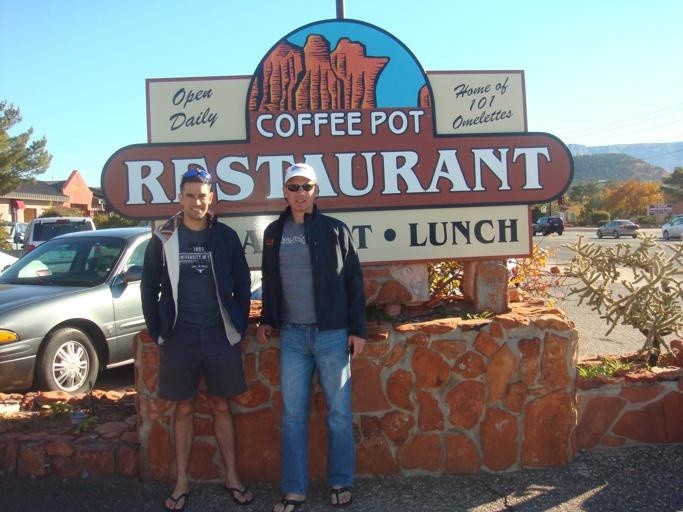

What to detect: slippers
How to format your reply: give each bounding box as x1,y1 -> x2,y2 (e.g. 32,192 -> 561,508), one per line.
270,493 -> 307,512
223,481 -> 256,505
329,484 -> 354,507
164,487 -> 192,511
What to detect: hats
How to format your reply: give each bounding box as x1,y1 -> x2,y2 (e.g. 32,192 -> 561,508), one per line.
283,164 -> 318,188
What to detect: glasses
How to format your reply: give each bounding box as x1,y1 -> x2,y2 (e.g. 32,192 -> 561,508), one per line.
284,183 -> 317,191
181,170 -> 212,180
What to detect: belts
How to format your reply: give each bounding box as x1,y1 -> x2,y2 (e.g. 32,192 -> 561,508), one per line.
278,319 -> 317,329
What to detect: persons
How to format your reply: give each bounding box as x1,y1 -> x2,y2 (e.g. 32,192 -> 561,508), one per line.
140,170 -> 255,512
255,163 -> 366,512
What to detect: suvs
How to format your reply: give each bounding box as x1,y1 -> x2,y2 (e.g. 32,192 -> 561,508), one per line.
532,215 -> 564,236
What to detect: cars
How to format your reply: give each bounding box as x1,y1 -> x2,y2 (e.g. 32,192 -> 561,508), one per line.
597,219 -> 640,239
427,259 -> 522,297
662,214 -> 683,242
0,213 -> 158,403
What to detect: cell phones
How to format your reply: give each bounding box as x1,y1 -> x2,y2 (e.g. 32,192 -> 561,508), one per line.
348,343 -> 354,354
